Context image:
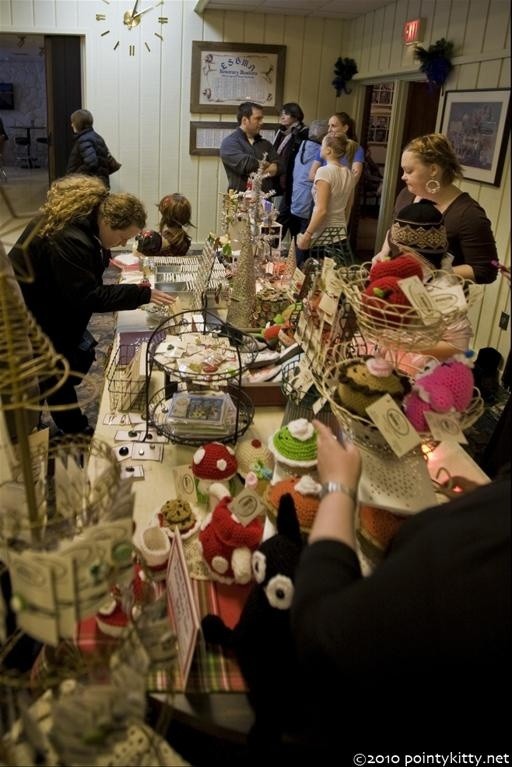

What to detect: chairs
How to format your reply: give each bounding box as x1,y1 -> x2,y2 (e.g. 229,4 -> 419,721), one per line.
12,136 -> 35,172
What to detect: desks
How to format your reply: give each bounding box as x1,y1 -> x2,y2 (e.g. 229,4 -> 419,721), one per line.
18,254 -> 495,767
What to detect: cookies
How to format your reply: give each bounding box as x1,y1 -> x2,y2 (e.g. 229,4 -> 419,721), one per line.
159,497 -> 196,534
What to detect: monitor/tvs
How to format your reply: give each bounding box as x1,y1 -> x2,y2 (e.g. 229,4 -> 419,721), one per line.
0,82 -> 15,110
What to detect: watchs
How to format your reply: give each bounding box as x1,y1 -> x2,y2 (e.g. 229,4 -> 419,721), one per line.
318,481 -> 356,501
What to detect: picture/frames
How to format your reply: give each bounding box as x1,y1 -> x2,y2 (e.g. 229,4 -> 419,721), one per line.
437,85 -> 512,187
188,121 -> 281,157
369,83 -> 394,108
189,40 -> 287,118
367,111 -> 390,145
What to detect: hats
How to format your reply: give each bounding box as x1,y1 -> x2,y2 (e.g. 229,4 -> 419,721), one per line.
192,419 -> 322,534
388,203 -> 448,256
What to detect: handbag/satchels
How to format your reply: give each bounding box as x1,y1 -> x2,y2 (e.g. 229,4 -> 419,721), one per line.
72,329 -> 96,385
107,153 -> 120,175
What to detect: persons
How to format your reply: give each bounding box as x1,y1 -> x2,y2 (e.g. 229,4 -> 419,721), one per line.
0,174 -> 176,445
220,102 -> 381,271
201,493 -> 308,706
372,134 -> 498,285
65,109 -> 122,269
291,419 -> 512,767
369,203 -> 474,378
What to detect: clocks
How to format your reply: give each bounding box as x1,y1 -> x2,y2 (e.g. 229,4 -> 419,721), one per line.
90,0 -> 170,59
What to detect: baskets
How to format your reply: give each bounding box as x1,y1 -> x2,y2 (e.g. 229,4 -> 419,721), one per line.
149,322 -> 258,381
106,344 -> 153,413
147,381 -> 255,446
337,262 -> 480,351
310,343 -> 485,457
281,360 -> 329,412
